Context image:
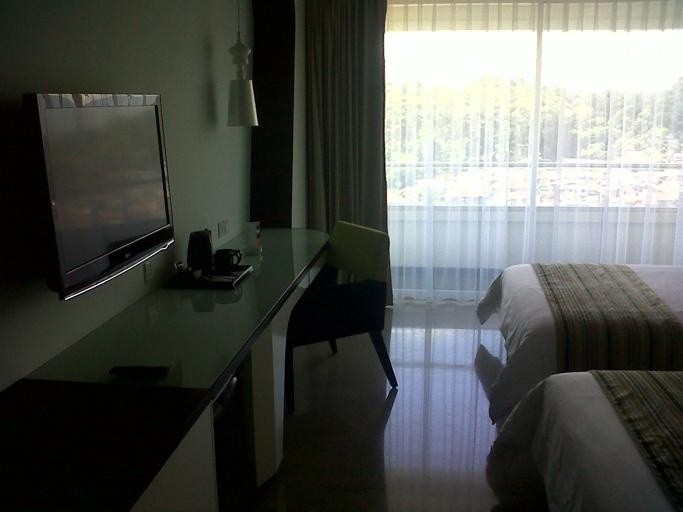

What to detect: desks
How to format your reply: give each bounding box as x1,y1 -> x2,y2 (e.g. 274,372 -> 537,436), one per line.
2,228 -> 330,512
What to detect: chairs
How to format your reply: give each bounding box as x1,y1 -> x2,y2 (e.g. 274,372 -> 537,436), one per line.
283,220 -> 398,415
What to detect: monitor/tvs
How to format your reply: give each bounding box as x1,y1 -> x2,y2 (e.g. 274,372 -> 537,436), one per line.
20,89 -> 175,302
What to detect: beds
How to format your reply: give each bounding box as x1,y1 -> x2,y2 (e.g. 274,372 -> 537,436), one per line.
473,264 -> 683,419
486,371 -> 683,512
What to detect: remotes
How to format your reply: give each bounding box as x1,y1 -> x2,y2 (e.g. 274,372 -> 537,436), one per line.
109,366 -> 172,379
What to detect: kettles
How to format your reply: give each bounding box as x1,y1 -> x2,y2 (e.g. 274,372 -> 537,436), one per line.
186,228 -> 212,271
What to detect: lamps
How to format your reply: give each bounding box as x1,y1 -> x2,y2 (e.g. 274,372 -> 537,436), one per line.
226,0 -> 260,126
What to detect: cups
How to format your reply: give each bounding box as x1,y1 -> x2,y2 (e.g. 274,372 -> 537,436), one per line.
213,248 -> 242,272
